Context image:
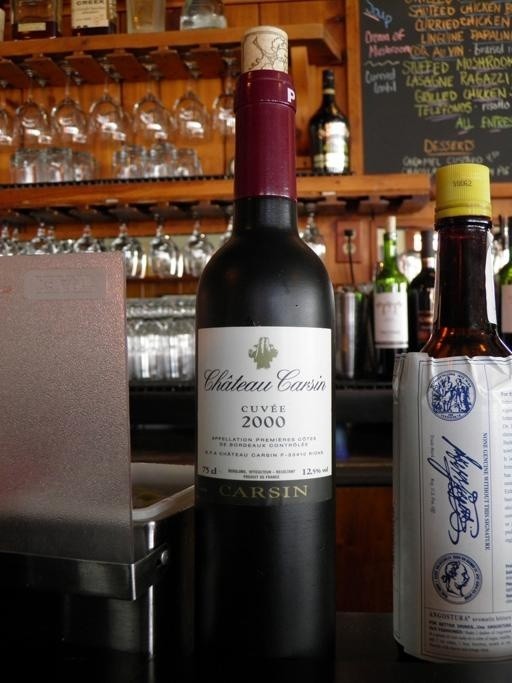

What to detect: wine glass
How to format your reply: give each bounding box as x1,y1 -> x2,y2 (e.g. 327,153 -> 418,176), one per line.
0,49 -> 239,186
0,204 -> 327,281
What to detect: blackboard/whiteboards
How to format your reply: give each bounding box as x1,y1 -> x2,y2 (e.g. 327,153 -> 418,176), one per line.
345,0 -> 512,200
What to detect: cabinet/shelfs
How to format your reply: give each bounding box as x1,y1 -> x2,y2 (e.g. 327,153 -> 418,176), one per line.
0,0 -> 512,388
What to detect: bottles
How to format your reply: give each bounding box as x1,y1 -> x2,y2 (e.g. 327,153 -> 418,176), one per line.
7,0 -> 63,41
128,294 -> 196,383
192,23 -> 340,681
126,0 -> 166,35
307,68 -> 351,177
410,227 -> 436,354
178,0 -> 228,31
367,214 -> 416,383
69,0 -> 119,37
496,215 -> 512,353
389,161 -> 512,682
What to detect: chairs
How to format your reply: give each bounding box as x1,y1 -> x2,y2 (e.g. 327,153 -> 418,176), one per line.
0,254 -> 198,681
